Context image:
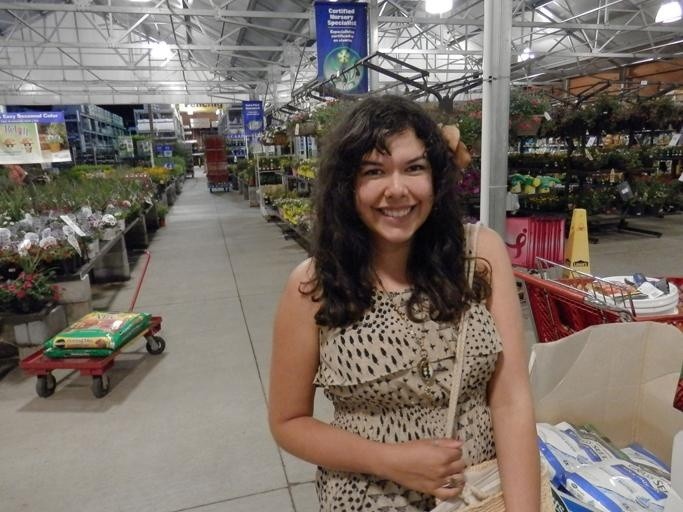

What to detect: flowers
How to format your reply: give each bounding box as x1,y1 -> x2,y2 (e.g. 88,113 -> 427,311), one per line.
0,272 -> 67,303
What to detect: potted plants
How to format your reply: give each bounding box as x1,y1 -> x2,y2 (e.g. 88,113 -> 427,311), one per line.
1,154 -> 186,270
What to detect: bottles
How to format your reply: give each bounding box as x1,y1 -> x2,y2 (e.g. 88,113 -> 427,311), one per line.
592,171 -> 595,185
595,170 -> 600,185
610,168 -> 615,182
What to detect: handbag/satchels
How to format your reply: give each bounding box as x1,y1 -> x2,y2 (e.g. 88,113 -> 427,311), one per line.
432,452 -> 555,512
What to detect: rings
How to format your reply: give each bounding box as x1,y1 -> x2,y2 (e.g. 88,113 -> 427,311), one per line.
444,475 -> 457,488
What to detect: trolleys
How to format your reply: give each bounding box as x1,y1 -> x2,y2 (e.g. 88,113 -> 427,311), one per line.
18,250 -> 165,398
514,257 -> 682,408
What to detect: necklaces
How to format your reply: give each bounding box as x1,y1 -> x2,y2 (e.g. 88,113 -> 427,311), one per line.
368,264 -> 434,382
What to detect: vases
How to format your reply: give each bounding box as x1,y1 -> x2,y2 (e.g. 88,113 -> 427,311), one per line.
15,301 -> 33,314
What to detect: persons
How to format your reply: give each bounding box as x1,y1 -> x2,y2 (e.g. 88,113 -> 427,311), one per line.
3,164 -> 27,185
268,93 -> 541,512
